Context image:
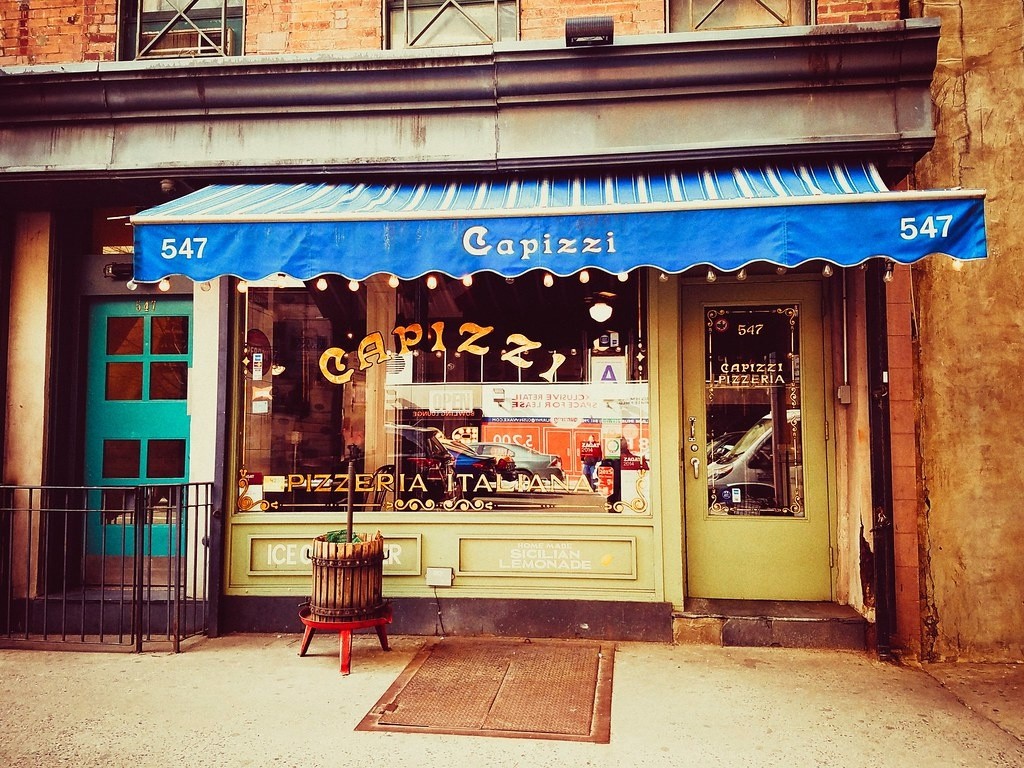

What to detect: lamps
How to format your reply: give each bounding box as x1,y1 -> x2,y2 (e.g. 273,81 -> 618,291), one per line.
882,259 -> 896,283
127,261 -> 869,294
589,298 -> 613,322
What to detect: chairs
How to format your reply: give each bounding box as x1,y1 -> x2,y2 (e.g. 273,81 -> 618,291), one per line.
362,464 -> 396,511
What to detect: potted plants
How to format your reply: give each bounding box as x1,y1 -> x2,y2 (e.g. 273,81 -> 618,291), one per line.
307,529 -> 389,622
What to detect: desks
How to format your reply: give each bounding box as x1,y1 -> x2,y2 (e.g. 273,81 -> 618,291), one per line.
274,482 -> 342,512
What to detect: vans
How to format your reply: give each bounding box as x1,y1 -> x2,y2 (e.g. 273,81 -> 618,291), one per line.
708,410 -> 803,507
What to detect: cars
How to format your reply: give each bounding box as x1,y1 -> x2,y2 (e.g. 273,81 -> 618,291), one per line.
461,443 -> 518,492
466,442 -> 565,493
705,429 -> 750,465
326,422 -> 463,511
439,439 -> 497,500
